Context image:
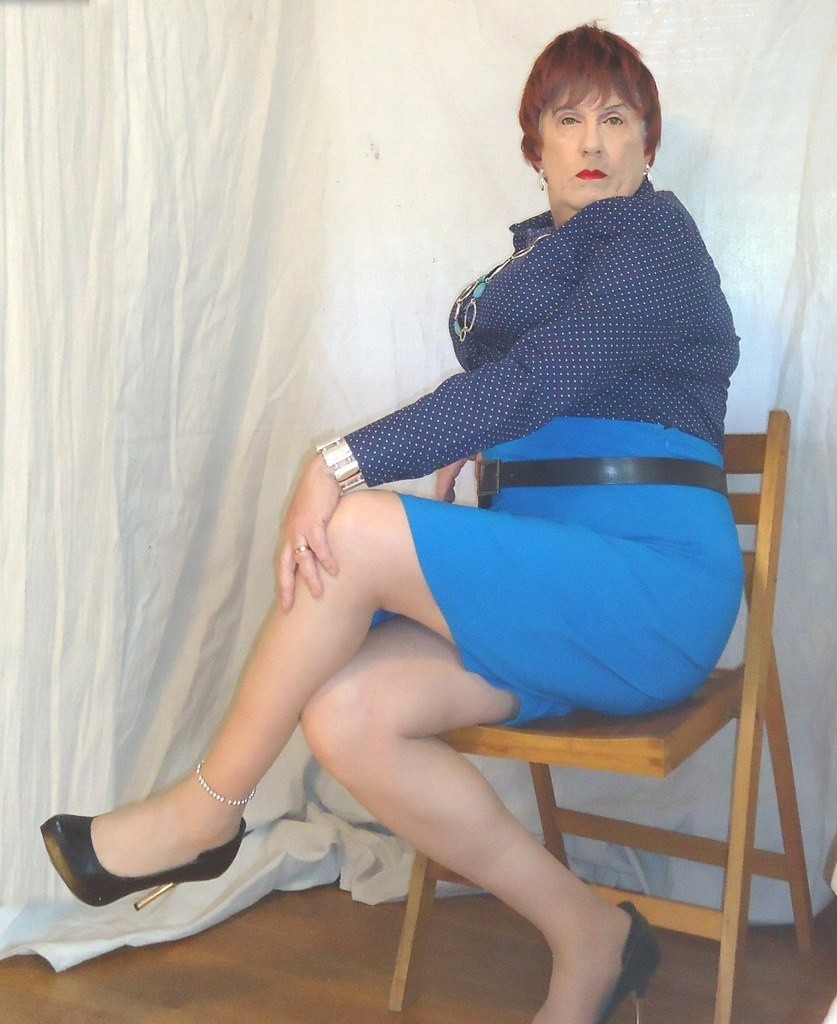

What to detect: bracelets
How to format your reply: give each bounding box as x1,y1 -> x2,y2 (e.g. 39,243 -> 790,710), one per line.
315,438 -> 366,493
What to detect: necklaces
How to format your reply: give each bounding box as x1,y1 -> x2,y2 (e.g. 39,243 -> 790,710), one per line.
451,229 -> 553,343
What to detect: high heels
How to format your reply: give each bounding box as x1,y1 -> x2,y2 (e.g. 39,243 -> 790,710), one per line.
599,901 -> 661,1024
42,813 -> 250,915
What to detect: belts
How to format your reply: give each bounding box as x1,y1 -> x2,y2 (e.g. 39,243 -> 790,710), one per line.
476,456 -> 727,495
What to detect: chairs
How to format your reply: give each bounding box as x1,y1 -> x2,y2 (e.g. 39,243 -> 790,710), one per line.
391,409 -> 818,1024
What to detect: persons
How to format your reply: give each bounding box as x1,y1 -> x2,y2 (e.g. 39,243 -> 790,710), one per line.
38,24 -> 746,1024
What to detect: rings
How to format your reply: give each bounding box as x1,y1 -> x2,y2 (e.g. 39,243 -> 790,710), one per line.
293,545 -> 310,556
